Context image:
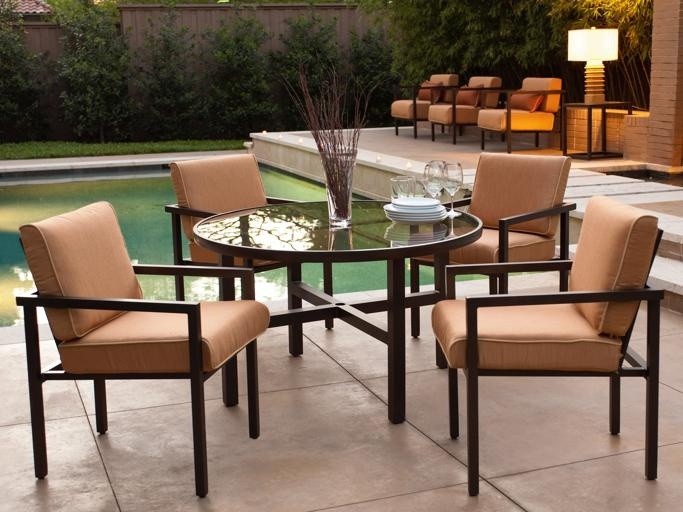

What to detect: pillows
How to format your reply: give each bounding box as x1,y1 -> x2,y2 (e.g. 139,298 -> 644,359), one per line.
456,83 -> 484,107
418,80 -> 443,104
511,94 -> 545,113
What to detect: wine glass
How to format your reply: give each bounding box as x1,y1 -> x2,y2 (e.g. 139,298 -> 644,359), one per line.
440,164 -> 463,218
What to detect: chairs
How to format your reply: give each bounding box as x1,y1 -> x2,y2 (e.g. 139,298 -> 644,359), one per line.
391,74 -> 459,139
428,77 -> 502,144
410,152 -> 577,338
15,200 -> 271,497
478,77 -> 565,154
431,195 -> 667,496
165,153 -> 333,330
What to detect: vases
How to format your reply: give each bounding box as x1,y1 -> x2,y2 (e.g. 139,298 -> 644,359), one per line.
320,151 -> 358,227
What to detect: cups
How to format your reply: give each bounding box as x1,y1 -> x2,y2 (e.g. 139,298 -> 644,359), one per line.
408,159 -> 446,200
388,176 -> 414,198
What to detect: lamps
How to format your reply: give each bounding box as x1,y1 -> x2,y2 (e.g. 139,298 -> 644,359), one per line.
567,26 -> 619,104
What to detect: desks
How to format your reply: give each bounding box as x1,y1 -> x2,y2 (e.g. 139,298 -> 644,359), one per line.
562,102 -> 632,160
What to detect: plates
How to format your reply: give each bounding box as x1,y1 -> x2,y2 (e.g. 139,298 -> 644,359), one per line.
382,198 -> 447,224
382,222 -> 447,246
